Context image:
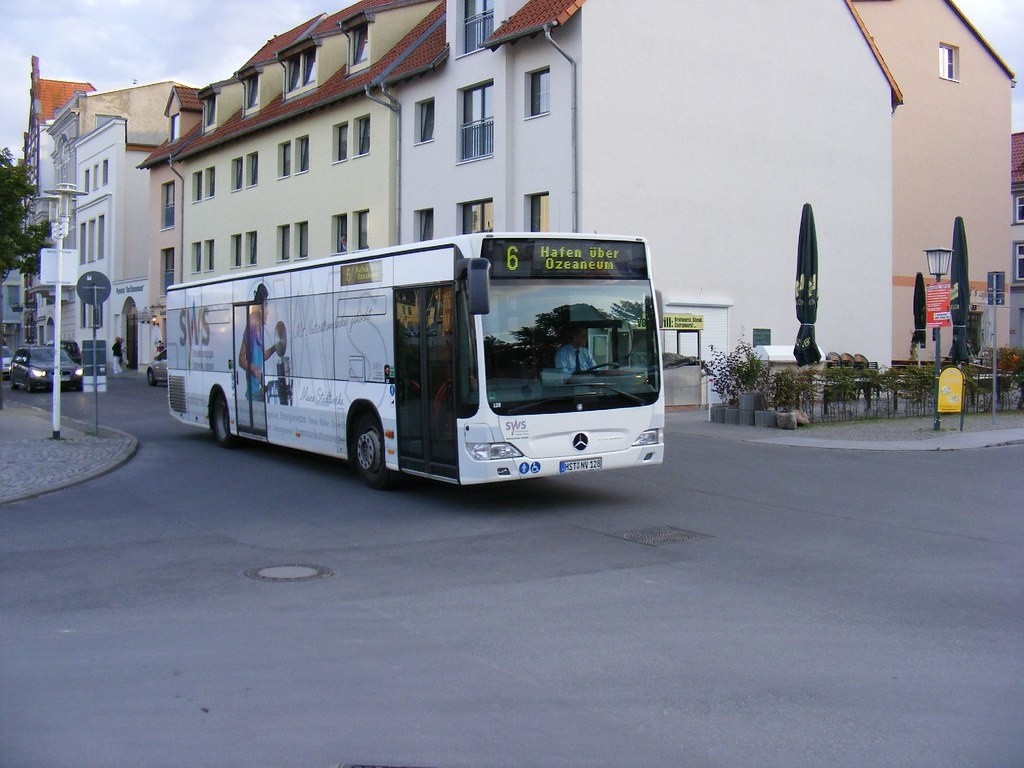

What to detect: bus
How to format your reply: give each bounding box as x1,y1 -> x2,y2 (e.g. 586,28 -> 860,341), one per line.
164,234 -> 666,492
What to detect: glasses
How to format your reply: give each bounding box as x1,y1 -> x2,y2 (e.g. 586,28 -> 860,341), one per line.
581,335 -> 588,338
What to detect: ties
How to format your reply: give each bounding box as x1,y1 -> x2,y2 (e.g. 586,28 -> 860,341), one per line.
575,349 -> 580,370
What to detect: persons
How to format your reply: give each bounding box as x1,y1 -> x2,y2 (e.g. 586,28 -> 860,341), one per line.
239,283 -> 276,402
554,327 -> 599,375
112,336 -> 126,375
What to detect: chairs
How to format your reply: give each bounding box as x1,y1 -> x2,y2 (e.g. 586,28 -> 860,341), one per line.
532,327 -> 566,380
825,352 -> 870,369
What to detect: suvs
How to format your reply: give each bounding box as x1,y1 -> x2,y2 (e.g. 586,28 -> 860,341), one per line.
9,344 -> 84,394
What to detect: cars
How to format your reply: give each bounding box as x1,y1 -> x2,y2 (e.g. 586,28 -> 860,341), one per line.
2,346 -> 15,378
147,345 -> 168,387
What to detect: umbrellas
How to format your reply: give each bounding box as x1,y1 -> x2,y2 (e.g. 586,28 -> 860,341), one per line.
911,272 -> 926,367
949,216 -> 971,370
793,203 -> 822,424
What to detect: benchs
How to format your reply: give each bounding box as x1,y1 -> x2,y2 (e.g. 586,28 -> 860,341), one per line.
823,363 -> 880,414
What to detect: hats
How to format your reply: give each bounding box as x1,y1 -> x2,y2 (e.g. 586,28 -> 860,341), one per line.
253,283 -> 268,303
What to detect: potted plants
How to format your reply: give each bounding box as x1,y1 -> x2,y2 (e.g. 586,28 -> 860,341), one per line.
702,337 -> 798,429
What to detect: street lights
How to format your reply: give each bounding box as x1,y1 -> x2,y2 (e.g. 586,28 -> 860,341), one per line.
925,247 -> 956,429
35,183 -> 90,436
